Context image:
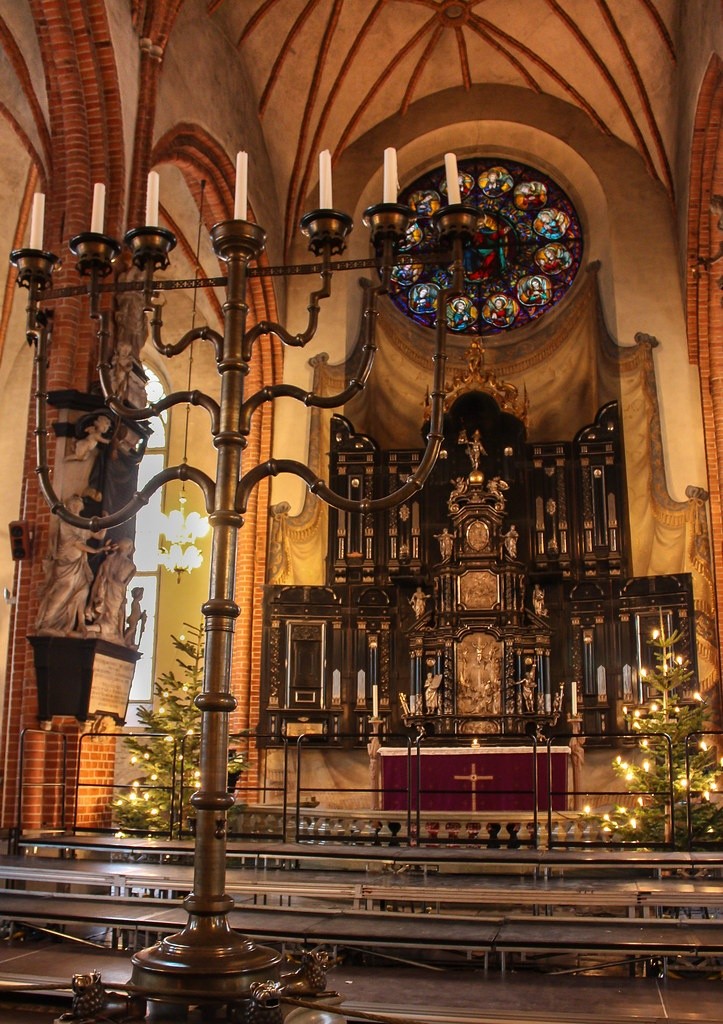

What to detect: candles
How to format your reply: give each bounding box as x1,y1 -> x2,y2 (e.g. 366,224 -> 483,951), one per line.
372,684 -> 378,718
572,681 -> 577,717
445,152 -> 462,205
144,171 -> 160,228
319,149 -> 333,210
234,148 -> 248,222
91,183 -> 106,235
383,147 -> 398,204
31,193 -> 45,251
471,739 -> 480,748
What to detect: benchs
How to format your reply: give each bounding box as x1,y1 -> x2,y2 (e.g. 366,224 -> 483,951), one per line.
0,834 -> 723,989
